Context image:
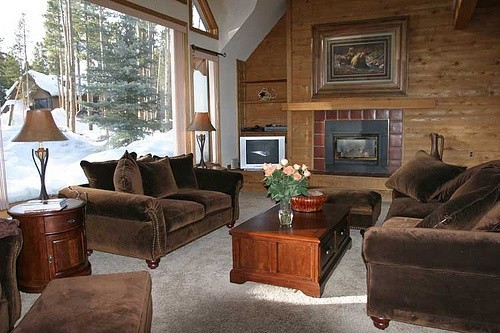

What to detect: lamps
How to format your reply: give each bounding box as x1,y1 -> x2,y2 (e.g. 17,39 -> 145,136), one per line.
185,112 -> 216,168
11,108 -> 69,199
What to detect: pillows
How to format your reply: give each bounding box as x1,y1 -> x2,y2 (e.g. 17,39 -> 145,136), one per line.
137,153 -> 154,162
427,160 -> 500,202
79,159 -> 118,190
155,153 -> 185,160
166,153 -> 198,188
140,157 -> 179,199
113,149 -> 144,194
413,162 -> 500,230
385,150 -> 467,203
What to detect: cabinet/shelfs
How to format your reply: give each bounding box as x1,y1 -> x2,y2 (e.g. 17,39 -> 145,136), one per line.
8,197 -> 93,293
239,77 -> 288,136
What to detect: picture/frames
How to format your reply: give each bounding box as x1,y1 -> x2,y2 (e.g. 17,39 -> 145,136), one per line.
310,14 -> 410,100
333,135 -> 379,161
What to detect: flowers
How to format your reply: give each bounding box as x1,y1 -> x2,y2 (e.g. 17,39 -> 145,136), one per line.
261,158 -> 311,199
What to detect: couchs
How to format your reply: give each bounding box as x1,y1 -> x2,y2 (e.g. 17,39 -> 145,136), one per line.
58,149 -> 244,269
362,189 -> 500,332
0,218 -> 22,332
325,190 -> 382,229
13,269 -> 153,333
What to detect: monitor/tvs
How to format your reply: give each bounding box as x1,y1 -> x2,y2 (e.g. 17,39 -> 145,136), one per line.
240,136 -> 285,171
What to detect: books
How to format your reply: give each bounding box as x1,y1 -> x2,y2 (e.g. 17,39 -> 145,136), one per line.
24,198 -> 68,213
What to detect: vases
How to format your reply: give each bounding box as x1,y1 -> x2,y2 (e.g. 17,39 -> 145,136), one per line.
278,199 -> 294,227
